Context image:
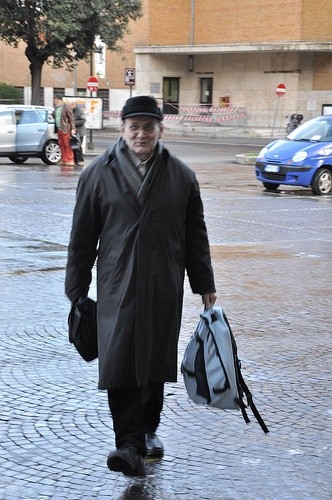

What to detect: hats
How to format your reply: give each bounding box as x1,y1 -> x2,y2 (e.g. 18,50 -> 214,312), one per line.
121,96 -> 164,121
67,297 -> 101,362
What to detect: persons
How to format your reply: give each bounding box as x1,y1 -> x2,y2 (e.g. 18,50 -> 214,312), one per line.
49,95 -> 76,166
289,114 -> 303,132
69,101 -> 86,166
64,96 -> 217,479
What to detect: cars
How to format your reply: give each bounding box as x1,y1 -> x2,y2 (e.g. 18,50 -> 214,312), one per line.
254,113 -> 332,196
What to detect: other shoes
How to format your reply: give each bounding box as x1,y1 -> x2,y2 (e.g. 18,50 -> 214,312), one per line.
56,159 -> 74,167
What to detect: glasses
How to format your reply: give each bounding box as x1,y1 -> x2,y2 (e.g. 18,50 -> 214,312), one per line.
126,123 -> 159,132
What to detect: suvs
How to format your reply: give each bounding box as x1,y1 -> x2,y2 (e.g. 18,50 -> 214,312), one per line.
0,103 -> 62,164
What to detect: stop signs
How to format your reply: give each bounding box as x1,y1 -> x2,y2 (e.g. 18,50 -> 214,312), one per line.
275,83 -> 287,97
86,76 -> 99,92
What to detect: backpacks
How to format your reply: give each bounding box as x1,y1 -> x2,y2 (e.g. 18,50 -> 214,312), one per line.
181,304 -> 246,411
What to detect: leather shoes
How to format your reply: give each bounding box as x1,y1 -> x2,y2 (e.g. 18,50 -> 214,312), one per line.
144,431 -> 164,456
107,447 -> 147,479
